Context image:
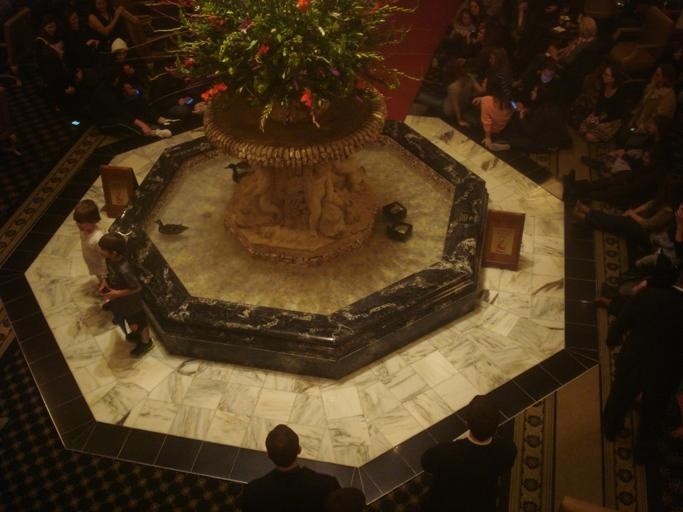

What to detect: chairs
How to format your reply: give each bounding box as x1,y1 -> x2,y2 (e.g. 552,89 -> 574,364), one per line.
527,120 -> 569,177
614,6 -> 674,85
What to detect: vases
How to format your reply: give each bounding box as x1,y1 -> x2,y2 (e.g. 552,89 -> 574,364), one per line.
204,88 -> 389,266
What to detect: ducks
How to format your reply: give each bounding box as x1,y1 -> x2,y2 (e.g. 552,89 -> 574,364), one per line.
224,163 -> 251,184
154,219 -> 188,234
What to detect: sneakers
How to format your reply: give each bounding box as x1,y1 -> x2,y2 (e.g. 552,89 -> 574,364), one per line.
130,339 -> 154,357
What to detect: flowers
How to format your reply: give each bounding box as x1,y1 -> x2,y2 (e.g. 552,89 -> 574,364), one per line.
100,0 -> 432,132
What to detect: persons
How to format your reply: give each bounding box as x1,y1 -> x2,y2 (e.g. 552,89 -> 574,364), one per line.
430,1 -> 683,512
3,2 -> 207,137
421,395 -> 519,512
240,424 -> 370,512
71,199 -> 156,357
